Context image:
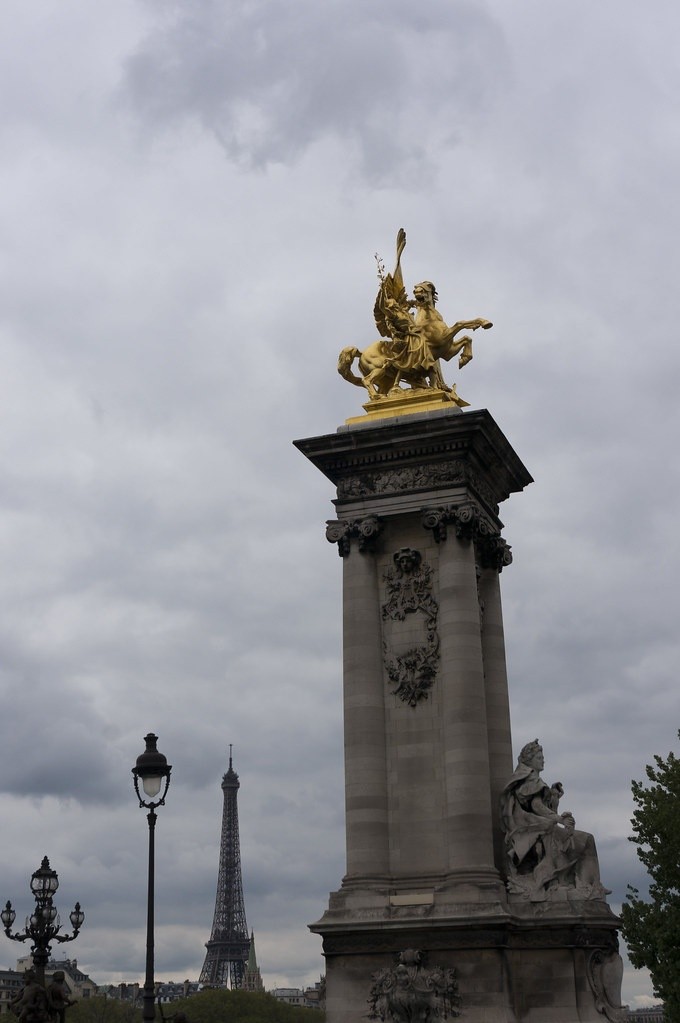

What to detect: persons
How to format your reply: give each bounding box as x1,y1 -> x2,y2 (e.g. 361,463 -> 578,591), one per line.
500,739 -> 611,898
362,286 -> 438,394
397,548 -> 417,580
9,970 -> 70,1023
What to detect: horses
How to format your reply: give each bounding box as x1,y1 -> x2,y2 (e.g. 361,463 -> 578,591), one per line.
337,280 -> 494,400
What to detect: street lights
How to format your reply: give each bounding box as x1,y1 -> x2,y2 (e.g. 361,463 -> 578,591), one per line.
131,733 -> 173,1023
0,854 -> 85,1022
